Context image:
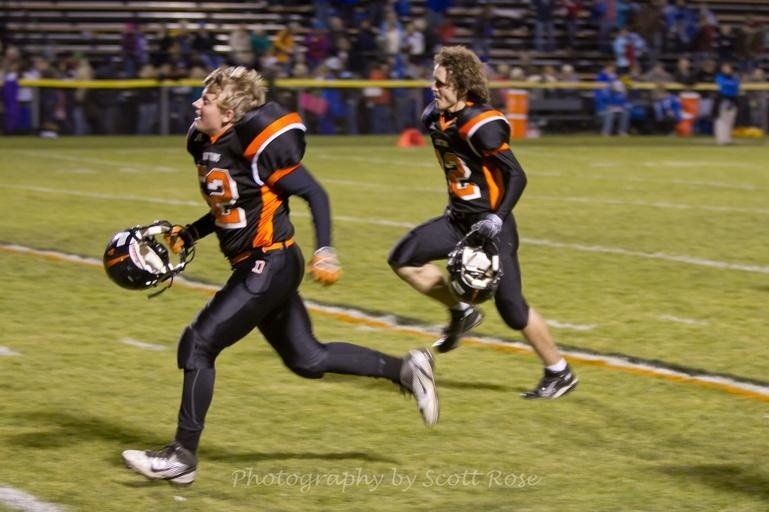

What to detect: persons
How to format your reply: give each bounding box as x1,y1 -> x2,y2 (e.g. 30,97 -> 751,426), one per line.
386,46 -> 579,400
122,64 -> 440,483
0,0 -> 769,145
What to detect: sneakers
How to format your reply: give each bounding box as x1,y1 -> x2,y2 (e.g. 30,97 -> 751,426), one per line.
522,364 -> 577,400
400,346 -> 439,429
434,301 -> 483,353
121,442 -> 196,488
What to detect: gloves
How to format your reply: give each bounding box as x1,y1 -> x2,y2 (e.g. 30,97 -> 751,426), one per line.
471,214 -> 503,240
164,224 -> 186,254
308,245 -> 341,286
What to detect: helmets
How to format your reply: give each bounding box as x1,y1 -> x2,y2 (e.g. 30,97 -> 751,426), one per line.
446,229 -> 504,305
103,220 -> 196,299
